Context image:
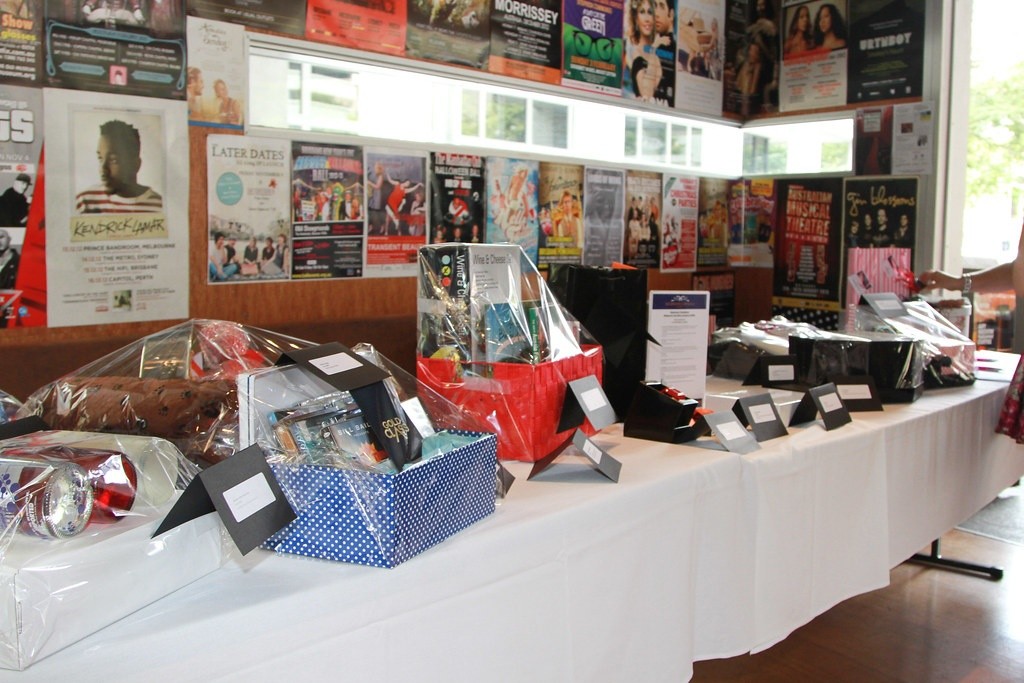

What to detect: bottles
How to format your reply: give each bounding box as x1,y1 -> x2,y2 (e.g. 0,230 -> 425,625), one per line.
0,431 -> 179,539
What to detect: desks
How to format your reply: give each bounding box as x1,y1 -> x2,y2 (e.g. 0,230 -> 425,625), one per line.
0,357 -> 1024,683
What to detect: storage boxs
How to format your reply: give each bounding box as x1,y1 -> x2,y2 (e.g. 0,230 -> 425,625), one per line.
258,426 -> 498,570
714,330 -> 975,401
418,244 -> 604,464
0,488 -> 221,671
